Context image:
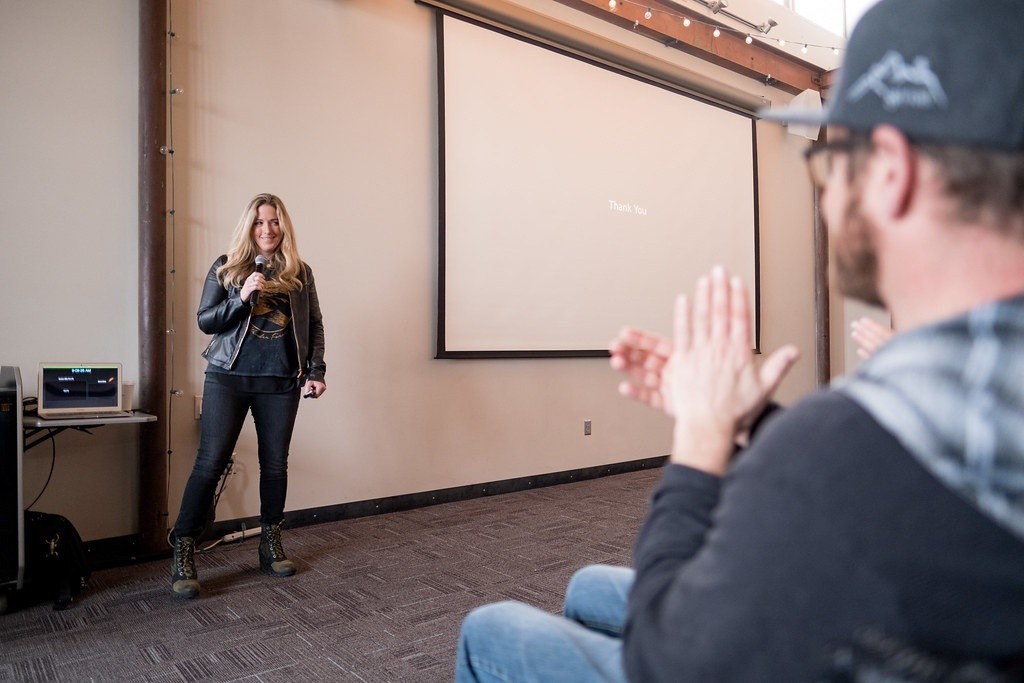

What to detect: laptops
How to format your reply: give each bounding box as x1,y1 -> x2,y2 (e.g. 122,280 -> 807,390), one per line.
38,363 -> 133,420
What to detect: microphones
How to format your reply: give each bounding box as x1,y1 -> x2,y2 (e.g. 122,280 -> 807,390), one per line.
250,255 -> 268,306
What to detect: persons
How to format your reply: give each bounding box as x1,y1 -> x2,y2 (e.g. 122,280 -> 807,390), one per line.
170,193 -> 326,598
457,0 -> 1024,683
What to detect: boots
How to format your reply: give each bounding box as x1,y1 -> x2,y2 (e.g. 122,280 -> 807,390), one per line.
258,518 -> 297,577
171,536 -> 201,600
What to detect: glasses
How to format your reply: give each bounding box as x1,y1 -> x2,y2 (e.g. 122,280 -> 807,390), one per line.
804,139 -> 872,189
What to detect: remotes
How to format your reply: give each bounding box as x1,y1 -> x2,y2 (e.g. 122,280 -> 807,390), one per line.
304,390 -> 316,398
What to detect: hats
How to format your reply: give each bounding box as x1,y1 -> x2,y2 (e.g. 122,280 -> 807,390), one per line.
754,0 -> 1024,151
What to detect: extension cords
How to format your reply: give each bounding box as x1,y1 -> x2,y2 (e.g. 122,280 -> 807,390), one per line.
222,528 -> 262,543
